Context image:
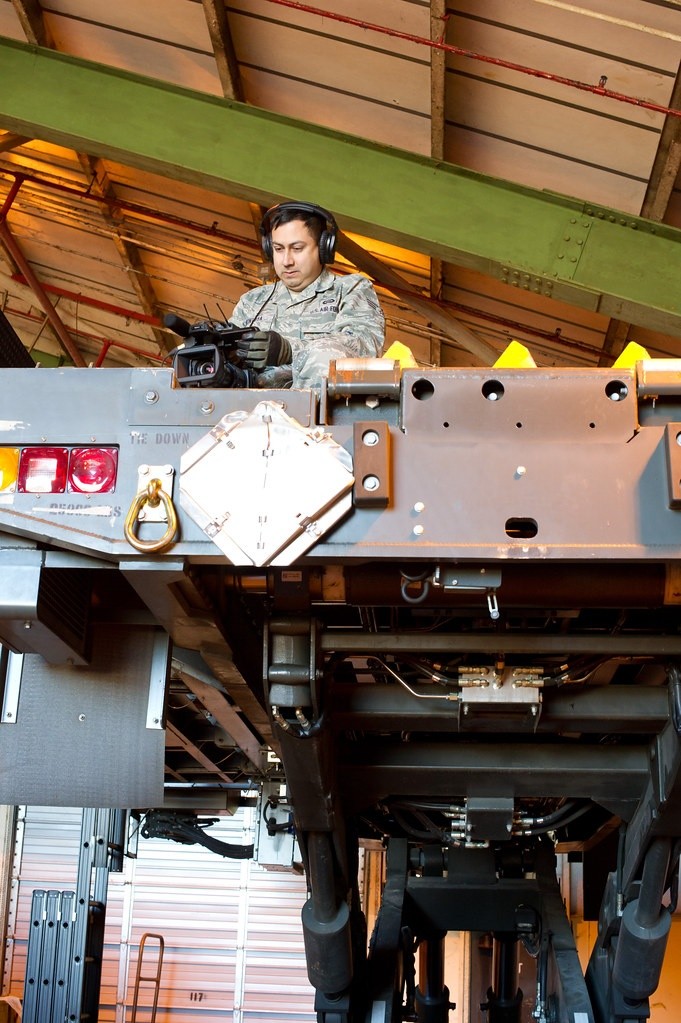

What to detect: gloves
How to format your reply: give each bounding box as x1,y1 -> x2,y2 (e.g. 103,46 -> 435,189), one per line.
236,331 -> 292,370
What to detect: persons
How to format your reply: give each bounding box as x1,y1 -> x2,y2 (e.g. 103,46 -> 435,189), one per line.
225,201 -> 386,389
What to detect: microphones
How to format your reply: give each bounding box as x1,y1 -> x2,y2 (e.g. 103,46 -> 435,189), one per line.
164,313 -> 192,338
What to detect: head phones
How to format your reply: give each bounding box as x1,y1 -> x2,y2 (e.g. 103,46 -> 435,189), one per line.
258,202 -> 339,264
